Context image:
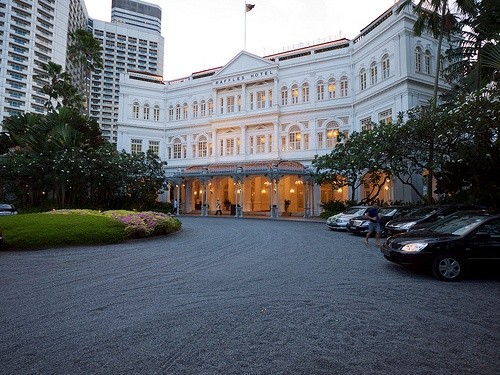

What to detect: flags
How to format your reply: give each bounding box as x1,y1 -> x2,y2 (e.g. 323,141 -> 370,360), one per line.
246,4 -> 255,12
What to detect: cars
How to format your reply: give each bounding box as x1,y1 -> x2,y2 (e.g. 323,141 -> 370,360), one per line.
349,206 -> 413,235
381,211 -> 500,282
384,204 -> 475,233
326,206 -> 373,232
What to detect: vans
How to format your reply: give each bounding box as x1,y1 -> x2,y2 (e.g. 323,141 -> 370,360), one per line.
0,204 -> 18,215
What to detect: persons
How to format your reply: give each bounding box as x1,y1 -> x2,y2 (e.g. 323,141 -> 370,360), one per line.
365,202 -> 382,247
214,199 -> 222,215
174,198 -> 184,214
196,198 -> 200,210
